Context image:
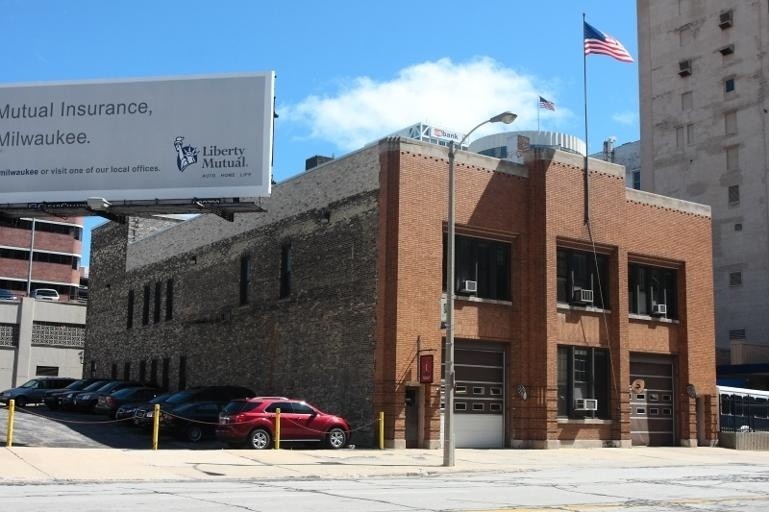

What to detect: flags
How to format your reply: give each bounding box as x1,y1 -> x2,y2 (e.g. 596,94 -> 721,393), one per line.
539,96 -> 555,112
585,21 -> 633,63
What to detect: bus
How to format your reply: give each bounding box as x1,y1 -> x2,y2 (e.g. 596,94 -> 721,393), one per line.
717,385 -> 769,432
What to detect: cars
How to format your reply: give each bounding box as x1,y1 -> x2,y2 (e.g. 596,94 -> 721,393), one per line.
94,386 -> 161,418
114,390 -> 175,426
0,289 -> 18,300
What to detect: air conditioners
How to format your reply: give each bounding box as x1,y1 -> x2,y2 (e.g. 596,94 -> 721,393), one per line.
575,398 -> 598,412
575,289 -> 594,304
653,304 -> 668,317
459,280 -> 478,294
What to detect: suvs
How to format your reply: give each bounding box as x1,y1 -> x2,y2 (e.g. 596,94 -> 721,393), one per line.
58,381 -> 108,410
216,395 -> 351,451
74,380 -> 143,412
40,381 -> 97,409
134,385 -> 258,445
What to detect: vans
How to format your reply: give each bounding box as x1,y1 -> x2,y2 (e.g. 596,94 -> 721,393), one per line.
31,288 -> 61,302
0,377 -> 78,406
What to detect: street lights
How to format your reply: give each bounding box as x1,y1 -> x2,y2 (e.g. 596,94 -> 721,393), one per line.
442,111 -> 518,469
